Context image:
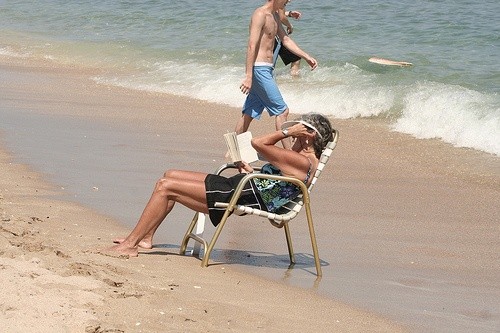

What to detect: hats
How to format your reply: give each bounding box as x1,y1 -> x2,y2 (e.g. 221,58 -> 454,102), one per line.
280,120 -> 323,139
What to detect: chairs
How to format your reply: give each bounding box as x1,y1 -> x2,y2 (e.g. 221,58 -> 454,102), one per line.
180,128 -> 339,275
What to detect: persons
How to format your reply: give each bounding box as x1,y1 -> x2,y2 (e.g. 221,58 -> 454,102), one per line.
96,113 -> 333,257
234,0 -> 317,150
273,8 -> 301,71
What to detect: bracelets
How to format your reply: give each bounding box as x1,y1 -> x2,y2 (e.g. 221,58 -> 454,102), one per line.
282,128 -> 289,138
288,11 -> 292,17
